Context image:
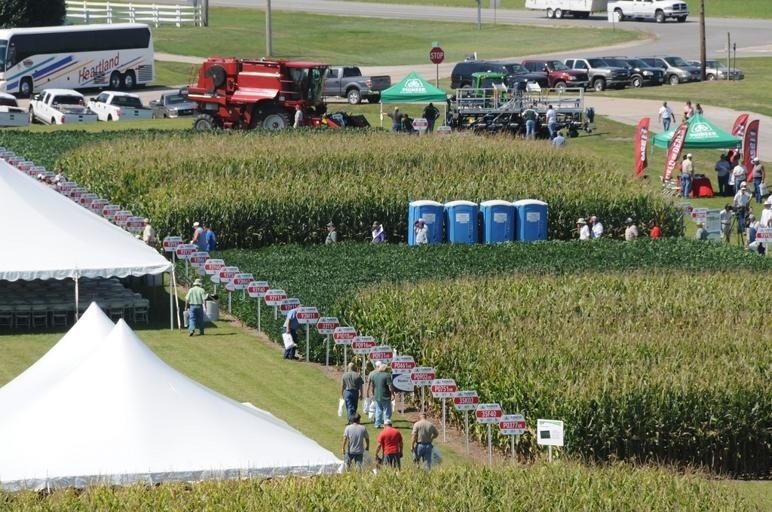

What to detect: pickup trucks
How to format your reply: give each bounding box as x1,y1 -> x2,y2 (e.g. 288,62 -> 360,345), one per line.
450,60 -> 548,95
320,65 -> 391,106
30,88 -> 98,126
150,91 -> 198,119
606,1 -> 689,24
89,91 -> 153,123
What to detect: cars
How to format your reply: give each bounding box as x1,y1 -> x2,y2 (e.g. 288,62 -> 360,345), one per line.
0,91 -> 30,129
685,59 -> 744,82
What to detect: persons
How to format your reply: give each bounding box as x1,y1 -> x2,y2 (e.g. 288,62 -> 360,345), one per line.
341,362 -> 363,425
375,419 -> 403,473
414,220 -> 429,246
422,103 -> 439,134
185,279 -> 207,336
381,107 -> 405,135
409,411 -> 439,472
189,222 -> 204,252
341,415 -> 370,474
371,221 -> 385,244
576,99 -> 772,257
402,113 -> 415,134
142,218 -> 155,248
366,360 -> 397,429
282,303 -> 304,360
325,221 -> 337,246
292,104 -> 303,130
521,104 -> 566,146
203,223 -> 216,253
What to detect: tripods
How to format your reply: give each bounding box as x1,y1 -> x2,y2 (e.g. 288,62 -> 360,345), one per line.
723,217 -> 745,247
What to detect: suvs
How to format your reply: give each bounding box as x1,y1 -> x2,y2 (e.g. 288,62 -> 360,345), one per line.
563,57 -> 631,93
520,59 -> 589,96
602,55 -> 666,88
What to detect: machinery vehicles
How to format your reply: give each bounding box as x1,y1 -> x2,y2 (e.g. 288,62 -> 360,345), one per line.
185,55 -> 372,132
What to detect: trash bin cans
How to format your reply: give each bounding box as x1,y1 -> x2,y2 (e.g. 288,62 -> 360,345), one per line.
444,201 -> 479,244
480,200 -> 516,244
203,299 -> 219,322
408,200 -> 445,246
513,199 -> 548,241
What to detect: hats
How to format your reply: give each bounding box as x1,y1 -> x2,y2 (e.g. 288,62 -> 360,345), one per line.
193,279 -> 202,285
384,419 -> 392,424
576,218 -> 586,224
192,222 -> 199,227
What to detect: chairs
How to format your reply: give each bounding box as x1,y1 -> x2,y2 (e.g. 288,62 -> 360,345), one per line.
0,278 -> 151,330
659,175 -> 683,198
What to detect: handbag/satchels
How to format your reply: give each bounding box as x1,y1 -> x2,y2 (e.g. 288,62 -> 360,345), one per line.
282,332 -> 298,350
338,399 -> 345,416
363,396 -> 377,422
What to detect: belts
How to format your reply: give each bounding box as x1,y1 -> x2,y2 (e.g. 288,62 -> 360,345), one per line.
416,442 -> 431,444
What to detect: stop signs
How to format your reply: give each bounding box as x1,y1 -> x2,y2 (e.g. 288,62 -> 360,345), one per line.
430,47 -> 445,64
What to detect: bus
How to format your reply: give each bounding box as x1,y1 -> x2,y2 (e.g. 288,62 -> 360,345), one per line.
0,23 -> 156,97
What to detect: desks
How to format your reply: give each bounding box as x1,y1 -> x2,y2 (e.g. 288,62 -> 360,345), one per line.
677,175 -> 714,198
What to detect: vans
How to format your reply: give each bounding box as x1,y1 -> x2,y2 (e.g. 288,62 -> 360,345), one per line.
636,56 -> 699,86
